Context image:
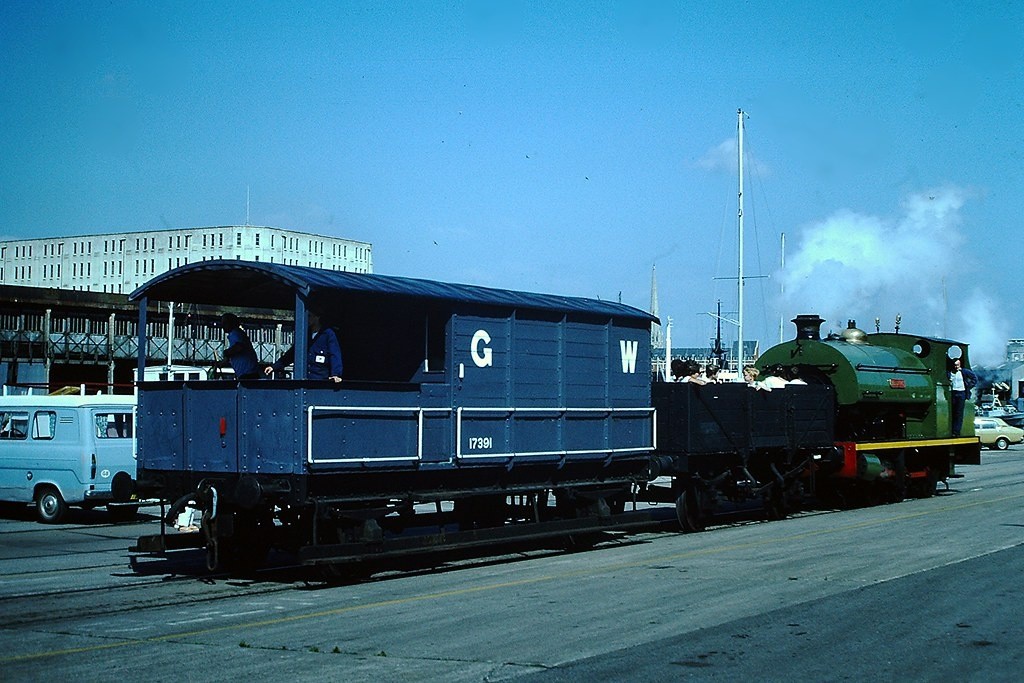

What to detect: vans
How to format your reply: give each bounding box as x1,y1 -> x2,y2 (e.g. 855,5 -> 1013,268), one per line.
0,393 -> 139,524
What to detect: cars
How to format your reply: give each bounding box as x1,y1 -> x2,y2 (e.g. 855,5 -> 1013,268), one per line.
972,416 -> 1024,450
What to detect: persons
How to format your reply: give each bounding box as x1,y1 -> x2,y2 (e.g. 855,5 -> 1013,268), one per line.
218,312 -> 259,380
264,299 -> 344,384
669,358 -> 808,391
948,357 -> 978,438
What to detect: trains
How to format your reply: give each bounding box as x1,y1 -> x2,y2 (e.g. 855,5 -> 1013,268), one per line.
107,257 -> 983,585
